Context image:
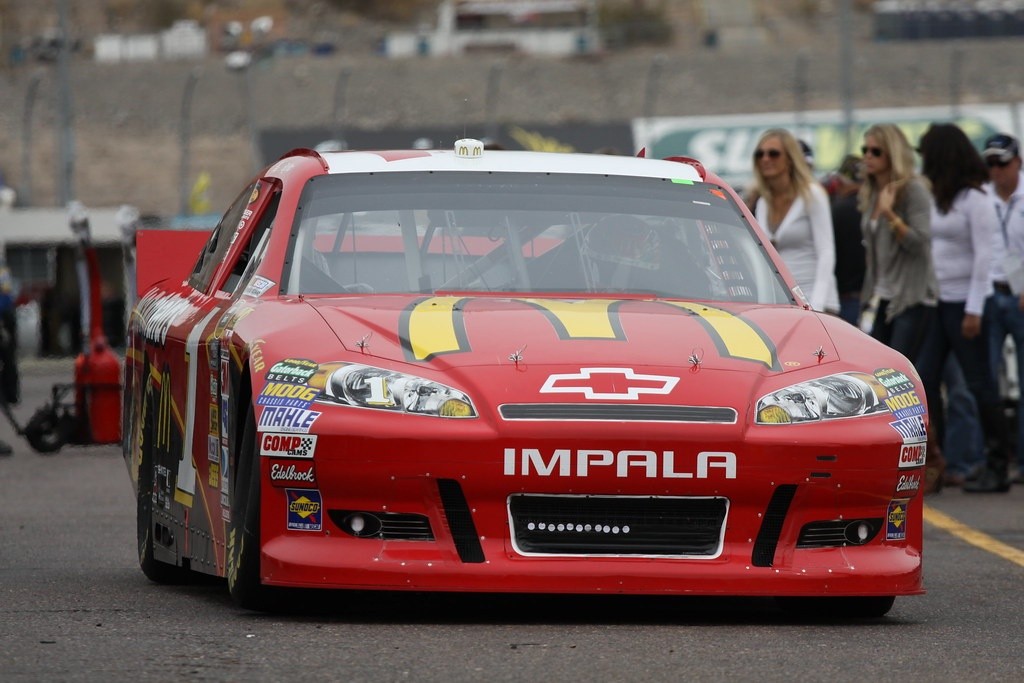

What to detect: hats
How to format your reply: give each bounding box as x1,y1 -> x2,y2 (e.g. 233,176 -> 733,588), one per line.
981,135 -> 1016,161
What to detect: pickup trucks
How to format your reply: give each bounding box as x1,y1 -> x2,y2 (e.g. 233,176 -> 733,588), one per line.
421,2 -> 600,62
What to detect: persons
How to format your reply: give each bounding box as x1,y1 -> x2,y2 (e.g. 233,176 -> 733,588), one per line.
752,126 -> 841,314
0,265 -> 23,458
740,120 -> 1024,492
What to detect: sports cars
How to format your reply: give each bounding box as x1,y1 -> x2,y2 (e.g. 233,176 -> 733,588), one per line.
115,139 -> 930,611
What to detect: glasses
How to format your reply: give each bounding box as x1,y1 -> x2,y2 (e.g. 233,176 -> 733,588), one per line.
862,147 -> 885,157
986,157 -> 1011,170
755,149 -> 786,160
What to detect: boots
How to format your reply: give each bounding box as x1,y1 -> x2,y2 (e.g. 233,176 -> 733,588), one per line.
923,414 -> 947,496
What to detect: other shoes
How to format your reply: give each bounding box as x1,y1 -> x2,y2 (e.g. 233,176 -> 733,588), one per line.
961,468 -> 1011,493
